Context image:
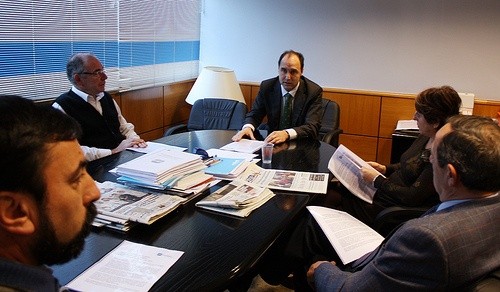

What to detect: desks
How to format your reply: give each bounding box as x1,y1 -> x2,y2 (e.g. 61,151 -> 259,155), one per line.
52,128 -> 338,292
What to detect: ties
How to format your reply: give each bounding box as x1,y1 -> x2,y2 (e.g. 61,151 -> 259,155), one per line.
352,203 -> 442,270
281,93 -> 293,129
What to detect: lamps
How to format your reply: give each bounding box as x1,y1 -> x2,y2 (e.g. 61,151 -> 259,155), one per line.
185,66 -> 247,107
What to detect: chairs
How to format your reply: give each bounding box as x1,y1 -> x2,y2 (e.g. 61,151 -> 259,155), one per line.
166,97 -> 248,136
255,98 -> 344,151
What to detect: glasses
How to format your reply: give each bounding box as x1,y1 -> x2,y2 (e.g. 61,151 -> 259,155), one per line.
72,68 -> 106,77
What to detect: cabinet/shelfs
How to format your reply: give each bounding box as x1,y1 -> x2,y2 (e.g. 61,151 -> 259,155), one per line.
389,128 -> 422,164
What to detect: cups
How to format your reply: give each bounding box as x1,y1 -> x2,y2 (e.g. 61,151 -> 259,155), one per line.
261,144 -> 273,163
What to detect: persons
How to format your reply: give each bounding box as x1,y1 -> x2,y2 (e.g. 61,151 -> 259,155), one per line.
325,84 -> 462,210
50,53 -> 149,162
268,141 -> 310,213
226,114 -> 500,292
230,50 -> 323,145
0,94 -> 102,292
